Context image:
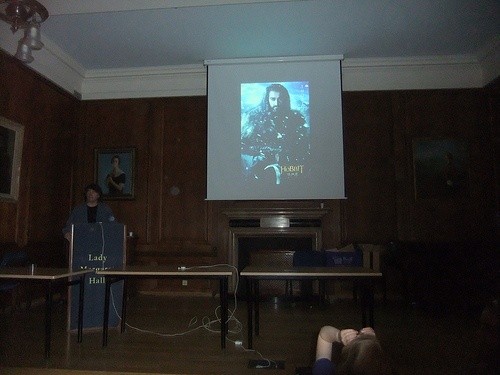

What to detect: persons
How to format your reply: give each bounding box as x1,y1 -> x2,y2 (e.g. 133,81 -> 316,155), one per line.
241,84 -> 309,183
312,326 -> 387,375
63,184 -> 115,243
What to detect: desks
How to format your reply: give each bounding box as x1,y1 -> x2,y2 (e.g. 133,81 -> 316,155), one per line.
239,266 -> 382,350
96,264 -> 235,351
0,267 -> 96,360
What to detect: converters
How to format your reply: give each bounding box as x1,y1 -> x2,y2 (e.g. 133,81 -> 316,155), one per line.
234,339 -> 244,345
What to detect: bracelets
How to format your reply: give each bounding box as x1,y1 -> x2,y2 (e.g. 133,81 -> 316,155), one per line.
336,330 -> 341,343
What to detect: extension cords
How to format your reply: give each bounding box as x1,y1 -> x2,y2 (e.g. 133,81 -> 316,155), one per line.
247,360 -> 285,369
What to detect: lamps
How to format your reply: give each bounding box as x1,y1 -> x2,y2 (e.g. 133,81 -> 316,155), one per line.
0,0 -> 49,64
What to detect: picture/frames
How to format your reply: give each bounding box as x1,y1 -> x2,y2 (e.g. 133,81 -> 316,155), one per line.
93,147 -> 135,201
